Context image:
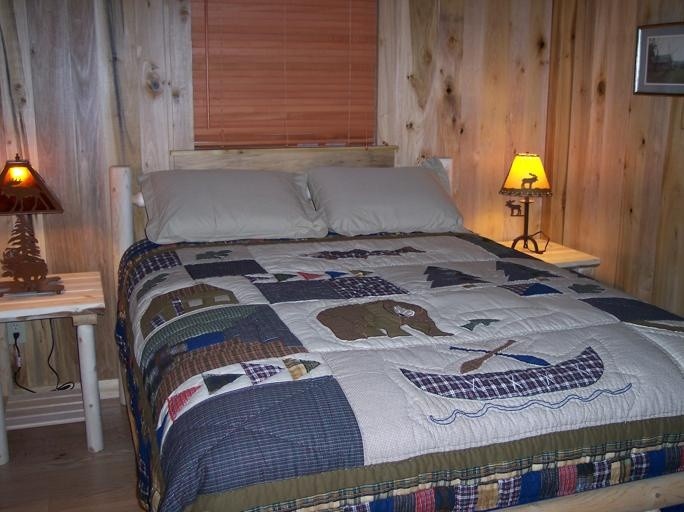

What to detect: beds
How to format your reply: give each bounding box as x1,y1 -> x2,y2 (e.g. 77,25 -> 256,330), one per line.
108,143 -> 684,511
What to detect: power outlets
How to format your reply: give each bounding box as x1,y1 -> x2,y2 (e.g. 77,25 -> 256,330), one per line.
6,322 -> 26,345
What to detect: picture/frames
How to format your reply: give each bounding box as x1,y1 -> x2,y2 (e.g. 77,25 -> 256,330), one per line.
633,21 -> 684,96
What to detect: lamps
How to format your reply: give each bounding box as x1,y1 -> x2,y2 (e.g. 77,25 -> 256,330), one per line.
498,151 -> 554,255
0,153 -> 64,298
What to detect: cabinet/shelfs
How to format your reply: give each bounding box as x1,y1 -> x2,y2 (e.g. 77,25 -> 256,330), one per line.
497,238 -> 601,281
0,271 -> 110,467
